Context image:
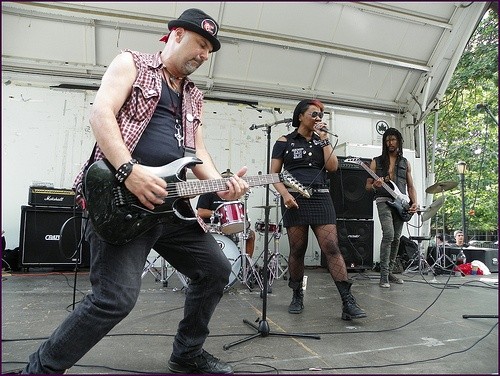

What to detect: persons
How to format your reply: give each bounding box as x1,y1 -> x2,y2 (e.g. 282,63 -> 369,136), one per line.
16,7 -> 249,376
271,100 -> 365,320
195,170 -> 255,258
366,128 -> 416,288
452,231 -> 464,248
434,234 -> 448,245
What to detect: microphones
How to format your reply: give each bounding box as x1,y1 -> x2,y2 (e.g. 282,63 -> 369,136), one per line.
320,127 -> 338,137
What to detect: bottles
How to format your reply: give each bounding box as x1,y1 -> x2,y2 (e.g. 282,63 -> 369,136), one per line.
456,251 -> 467,266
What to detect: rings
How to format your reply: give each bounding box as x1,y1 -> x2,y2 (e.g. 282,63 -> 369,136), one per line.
285,205 -> 288,208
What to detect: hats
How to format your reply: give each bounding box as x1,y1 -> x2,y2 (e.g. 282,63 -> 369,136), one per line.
292,99 -> 324,127
168,8 -> 221,52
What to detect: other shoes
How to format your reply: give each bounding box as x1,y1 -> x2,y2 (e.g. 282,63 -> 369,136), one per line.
379,275 -> 390,288
389,273 -> 403,283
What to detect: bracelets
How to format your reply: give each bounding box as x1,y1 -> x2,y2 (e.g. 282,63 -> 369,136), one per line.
321,139 -> 330,147
371,184 -> 377,191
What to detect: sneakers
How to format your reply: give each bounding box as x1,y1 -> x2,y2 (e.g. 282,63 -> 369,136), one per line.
169,349 -> 234,375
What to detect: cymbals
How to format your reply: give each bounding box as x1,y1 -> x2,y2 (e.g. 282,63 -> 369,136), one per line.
421,195 -> 445,222
252,206 -> 281,208
425,181 -> 459,193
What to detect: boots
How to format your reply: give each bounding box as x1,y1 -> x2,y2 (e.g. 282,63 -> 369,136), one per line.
334,279 -> 367,320
288,276 -> 304,314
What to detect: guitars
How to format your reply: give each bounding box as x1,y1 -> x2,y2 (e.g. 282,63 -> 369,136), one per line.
344,158 -> 414,222
85,156 -> 310,247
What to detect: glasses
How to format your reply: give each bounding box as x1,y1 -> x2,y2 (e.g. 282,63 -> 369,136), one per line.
458,235 -> 463,236
307,111 -> 323,118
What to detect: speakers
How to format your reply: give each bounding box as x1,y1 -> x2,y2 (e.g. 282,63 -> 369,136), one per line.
319,155 -> 375,269
20,206 -> 86,269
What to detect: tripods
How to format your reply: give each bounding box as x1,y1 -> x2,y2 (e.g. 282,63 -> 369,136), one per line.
231,172 -> 289,298
141,255 -> 189,290
223,117 -> 322,350
404,188 -> 466,276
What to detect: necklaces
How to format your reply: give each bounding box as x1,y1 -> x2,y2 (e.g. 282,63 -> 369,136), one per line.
162,69 -> 183,147
163,64 -> 182,89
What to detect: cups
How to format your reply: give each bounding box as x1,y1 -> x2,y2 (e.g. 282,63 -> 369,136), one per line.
302,275 -> 308,290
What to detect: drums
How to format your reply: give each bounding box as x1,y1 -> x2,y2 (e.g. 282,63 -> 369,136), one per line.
255,219 -> 282,236
176,231 -> 242,293
214,201 -> 251,236
